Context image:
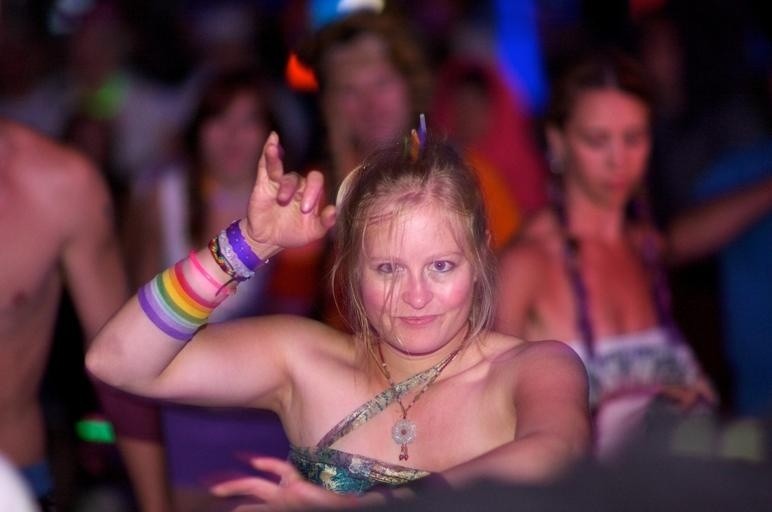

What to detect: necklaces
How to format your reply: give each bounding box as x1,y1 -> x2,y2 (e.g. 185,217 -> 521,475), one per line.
374,319 -> 473,462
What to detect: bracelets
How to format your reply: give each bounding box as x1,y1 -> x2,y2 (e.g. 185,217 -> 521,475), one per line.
137,217 -> 270,342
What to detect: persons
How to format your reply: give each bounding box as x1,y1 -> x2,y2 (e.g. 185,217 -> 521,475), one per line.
663,145 -> 772,413
0,113 -> 169,512
275,8 -> 520,333
491,62 -> 716,453
84,107 -> 590,512
120,66 -> 324,485
